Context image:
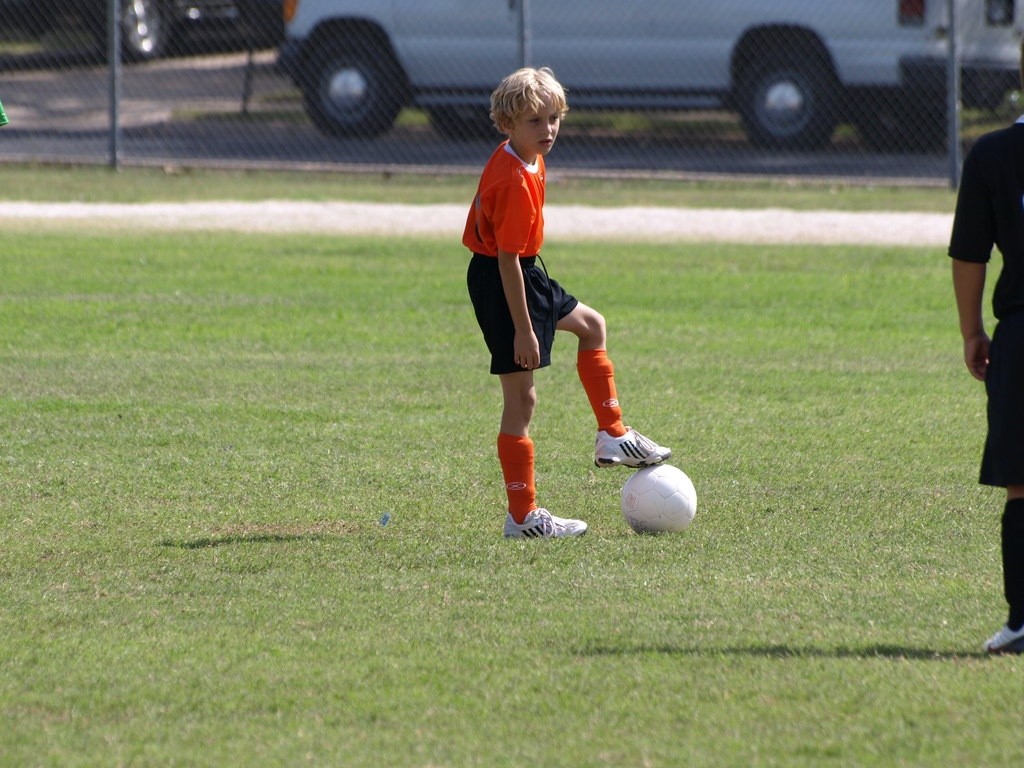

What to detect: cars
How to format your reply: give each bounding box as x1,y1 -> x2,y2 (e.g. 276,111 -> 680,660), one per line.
0,1 -> 283,64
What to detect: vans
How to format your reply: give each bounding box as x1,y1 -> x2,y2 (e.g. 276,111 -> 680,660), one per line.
270,0 -> 1024,158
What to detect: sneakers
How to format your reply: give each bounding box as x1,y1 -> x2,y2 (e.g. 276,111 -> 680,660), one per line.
595,426 -> 672,468
504,508 -> 587,540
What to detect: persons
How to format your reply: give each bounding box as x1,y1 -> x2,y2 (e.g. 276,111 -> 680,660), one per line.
461,67 -> 672,540
948,114 -> 1024,654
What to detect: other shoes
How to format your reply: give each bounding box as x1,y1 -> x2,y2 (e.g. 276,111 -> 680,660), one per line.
985,623 -> 1024,652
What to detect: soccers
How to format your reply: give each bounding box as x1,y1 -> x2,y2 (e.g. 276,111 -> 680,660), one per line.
616,462 -> 700,539
